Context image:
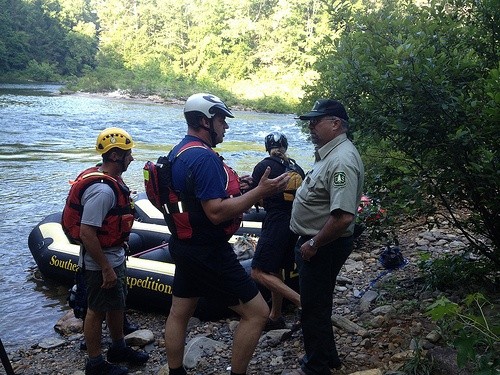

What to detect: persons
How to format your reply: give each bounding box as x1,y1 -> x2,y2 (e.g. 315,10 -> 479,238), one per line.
251,131 -> 306,331
289,99 -> 365,375
60,126 -> 149,375
143,91 -> 294,375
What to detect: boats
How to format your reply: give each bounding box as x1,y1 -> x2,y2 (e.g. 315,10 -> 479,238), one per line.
357,193 -> 386,225
27,211 -> 273,322
133,190 -> 267,237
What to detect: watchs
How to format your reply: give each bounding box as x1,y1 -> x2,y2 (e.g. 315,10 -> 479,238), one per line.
309,238 -> 317,249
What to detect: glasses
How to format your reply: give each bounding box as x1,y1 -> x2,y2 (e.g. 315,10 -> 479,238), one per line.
310,118 -> 337,125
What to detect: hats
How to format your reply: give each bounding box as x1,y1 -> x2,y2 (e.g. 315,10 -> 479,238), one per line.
300,99 -> 348,121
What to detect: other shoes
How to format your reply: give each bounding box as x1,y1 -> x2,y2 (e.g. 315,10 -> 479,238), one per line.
287,367 -> 307,375
263,317 -> 287,331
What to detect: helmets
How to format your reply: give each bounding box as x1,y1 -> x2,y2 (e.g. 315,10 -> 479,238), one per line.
95,128 -> 135,155
264,132 -> 288,152
183,93 -> 235,121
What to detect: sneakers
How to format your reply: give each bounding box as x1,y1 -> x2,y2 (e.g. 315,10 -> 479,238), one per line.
84,358 -> 128,375
106,346 -> 150,365
